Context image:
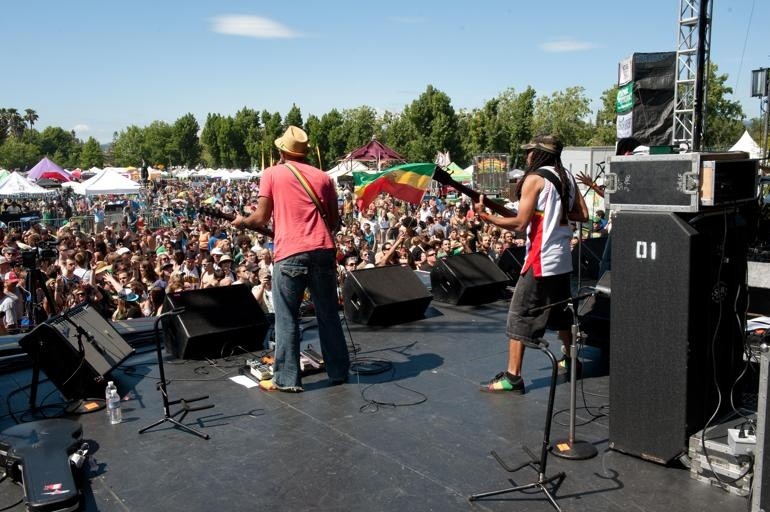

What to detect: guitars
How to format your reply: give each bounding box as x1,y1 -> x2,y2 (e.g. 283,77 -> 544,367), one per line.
196,203 -> 344,261
431,163 -> 527,240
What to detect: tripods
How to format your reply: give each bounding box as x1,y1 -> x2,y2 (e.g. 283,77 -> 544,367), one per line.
137,318 -> 212,442
465,351 -> 568,512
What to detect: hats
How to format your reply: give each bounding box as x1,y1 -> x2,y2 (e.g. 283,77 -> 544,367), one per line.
118,288 -> 139,301
211,248 -> 224,256
119,247 -> 130,256
6,247 -> 16,254
521,133 -> 563,156
218,255 -> 233,266
185,254 -> 197,261
336,231 -> 344,236
357,230 -> 363,237
4,272 -> 18,284
160,263 -> 173,271
275,126 -> 310,158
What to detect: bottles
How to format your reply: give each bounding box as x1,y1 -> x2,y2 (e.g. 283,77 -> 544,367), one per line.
105,381 -> 122,425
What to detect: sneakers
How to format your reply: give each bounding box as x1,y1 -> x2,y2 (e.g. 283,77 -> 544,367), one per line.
258,379 -> 280,392
479,371 -> 525,395
556,356 -> 582,371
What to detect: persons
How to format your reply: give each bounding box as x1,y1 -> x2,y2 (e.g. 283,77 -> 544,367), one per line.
574,137 -> 642,277
230,124 -> 353,395
1,165 -> 611,335
469,133 -> 589,395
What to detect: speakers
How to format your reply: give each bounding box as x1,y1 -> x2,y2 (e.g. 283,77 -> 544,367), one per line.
606,210 -> 749,468
496,246 -> 527,284
19,300 -> 137,404
340,263 -> 434,328
158,282 -> 271,363
429,250 -> 512,307
